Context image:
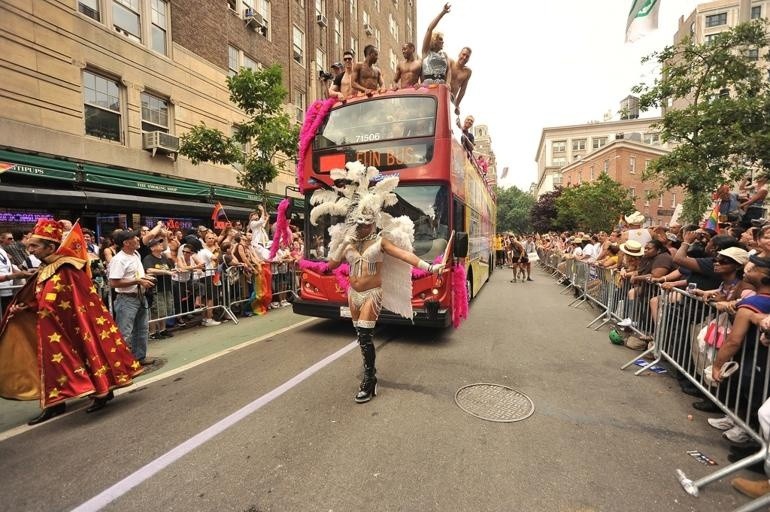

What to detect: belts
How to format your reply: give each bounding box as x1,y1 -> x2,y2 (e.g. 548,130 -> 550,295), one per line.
121,293 -> 144,297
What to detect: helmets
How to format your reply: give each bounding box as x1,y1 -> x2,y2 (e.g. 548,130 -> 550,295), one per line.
609,327 -> 624,344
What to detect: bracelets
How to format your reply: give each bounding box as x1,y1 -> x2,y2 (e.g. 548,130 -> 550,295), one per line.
428,264 -> 433,274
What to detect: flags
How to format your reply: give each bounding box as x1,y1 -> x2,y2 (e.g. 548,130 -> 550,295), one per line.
625,0 -> 661,41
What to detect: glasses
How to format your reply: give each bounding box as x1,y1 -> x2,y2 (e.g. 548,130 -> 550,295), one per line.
712,257 -> 733,265
182,250 -> 193,254
344,57 -> 352,61
4,236 -> 14,240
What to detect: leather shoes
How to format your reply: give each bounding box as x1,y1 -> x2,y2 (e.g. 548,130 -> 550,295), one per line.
29,402 -> 66,424
728,446 -> 766,474
693,400 -> 724,412
731,478 -> 770,499
86,391 -> 114,413
639,335 -> 653,340
684,385 -> 716,397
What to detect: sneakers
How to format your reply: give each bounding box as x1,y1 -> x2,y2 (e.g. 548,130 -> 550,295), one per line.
267,299 -> 291,310
616,317 -> 640,327
158,330 -> 173,337
721,424 -> 759,443
140,359 -> 154,364
510,275 -> 534,282
708,415 -> 737,431
150,332 -> 166,339
193,302 -> 205,308
175,320 -> 191,328
201,317 -> 221,327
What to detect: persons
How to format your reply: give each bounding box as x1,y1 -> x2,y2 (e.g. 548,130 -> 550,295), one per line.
284,197 -> 451,404
318,2 -> 493,179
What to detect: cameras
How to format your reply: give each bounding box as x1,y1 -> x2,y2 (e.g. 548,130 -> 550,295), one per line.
202,264 -> 207,272
142,275 -> 159,283
318,69 -> 333,81
686,282 -> 698,293
695,233 -> 704,240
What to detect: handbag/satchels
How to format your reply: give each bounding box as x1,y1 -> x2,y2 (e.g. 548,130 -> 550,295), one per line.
704,322 -> 732,350
226,265 -> 240,286
522,253 -> 529,262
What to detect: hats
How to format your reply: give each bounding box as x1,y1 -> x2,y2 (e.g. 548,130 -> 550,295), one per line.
619,240 -> 645,256
31,218 -> 65,244
331,62 -> 344,69
665,232 -> 678,242
146,238 -> 163,248
748,253 -> 770,267
717,247 -> 750,265
116,229 -> 139,243
571,235 -> 591,245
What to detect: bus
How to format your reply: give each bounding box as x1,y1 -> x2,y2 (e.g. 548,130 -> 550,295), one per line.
281,83 -> 498,329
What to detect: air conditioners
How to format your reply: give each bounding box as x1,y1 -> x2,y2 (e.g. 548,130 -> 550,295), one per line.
316,15 -> 329,27
242,7 -> 264,29
364,24 -> 373,35
142,130 -> 180,162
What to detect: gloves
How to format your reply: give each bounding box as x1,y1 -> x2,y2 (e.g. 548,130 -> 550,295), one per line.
428,264 -> 448,274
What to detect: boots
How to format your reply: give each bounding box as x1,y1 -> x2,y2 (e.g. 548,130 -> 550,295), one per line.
352,318 -> 377,403
